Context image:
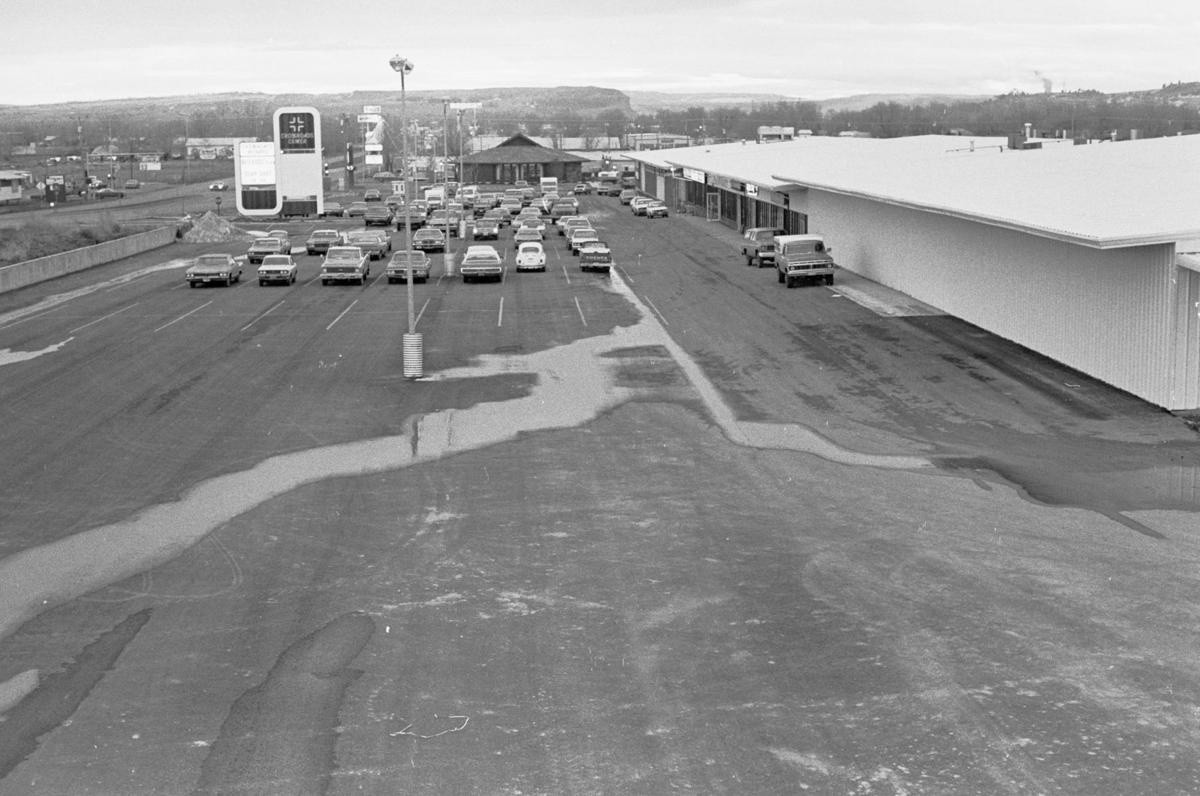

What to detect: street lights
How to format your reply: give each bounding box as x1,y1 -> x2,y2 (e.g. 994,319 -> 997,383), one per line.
440,93 -> 456,280
390,54 -> 425,380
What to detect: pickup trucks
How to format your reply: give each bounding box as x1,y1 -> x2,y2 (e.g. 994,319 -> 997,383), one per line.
579,241 -> 612,274
317,245 -> 371,287
771,233 -> 835,286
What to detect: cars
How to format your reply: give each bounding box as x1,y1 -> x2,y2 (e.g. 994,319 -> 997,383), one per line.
305,229 -> 345,255
514,241 -> 546,273
347,229 -> 391,262
257,254 -> 298,286
94,188 -> 124,198
383,195 -> 405,209
125,178 -> 139,189
396,164 -> 654,255
738,226 -> 792,269
348,201 -> 369,218
646,200 -> 669,220
459,245 -> 503,282
363,204 -> 393,225
247,228 -> 293,265
319,202 -> 345,218
363,189 -> 382,202
185,253 -> 245,288
209,180 -> 229,192
382,249 -> 431,282
